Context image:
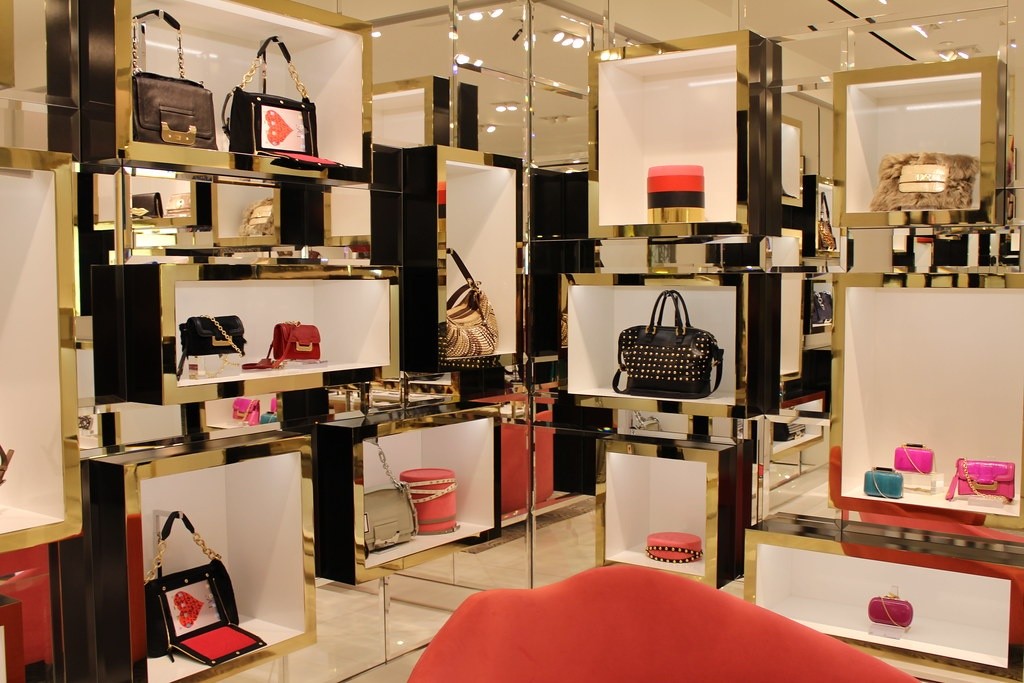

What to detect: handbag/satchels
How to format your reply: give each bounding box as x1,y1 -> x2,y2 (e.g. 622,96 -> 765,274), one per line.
132,8 -> 218,150
240,197 -> 275,235
446,249 -> 498,357
865,467 -> 903,498
869,594 -> 913,632
814,290 -> 833,324
364,444 -> 418,552
645,532 -> 704,564
817,192 -> 837,250
179,316 -> 246,366
143,512 -> 237,656
870,153 -> 978,209
233,398 -> 261,425
946,458 -> 1015,505
164,193 -> 191,218
132,192 -> 163,219
222,36 -> 319,158
894,443 -> 936,475
612,290 -> 723,400
242,323 -> 320,369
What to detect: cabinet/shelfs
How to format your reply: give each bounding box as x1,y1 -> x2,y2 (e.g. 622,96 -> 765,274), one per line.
1,2 -> 1024,683
